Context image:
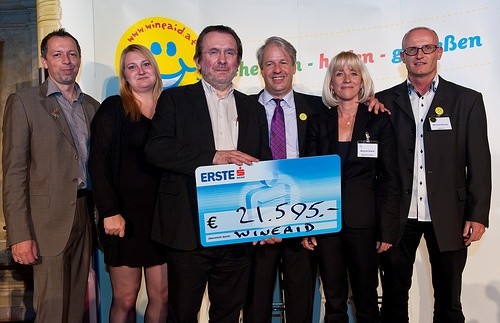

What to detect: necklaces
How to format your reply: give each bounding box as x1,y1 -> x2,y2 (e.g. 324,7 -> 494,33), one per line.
338,112 -> 356,126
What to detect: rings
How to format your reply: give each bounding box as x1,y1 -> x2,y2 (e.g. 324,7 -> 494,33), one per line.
384,248 -> 388,251
18,260 -> 23,263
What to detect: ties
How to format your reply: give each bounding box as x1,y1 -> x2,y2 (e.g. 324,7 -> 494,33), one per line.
270,99 -> 287,160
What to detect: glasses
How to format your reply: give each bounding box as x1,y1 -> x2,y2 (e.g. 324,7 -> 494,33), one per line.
402,44 -> 439,56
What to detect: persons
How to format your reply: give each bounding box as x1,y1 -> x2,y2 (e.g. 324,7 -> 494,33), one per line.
88,44 -> 169,323
243,37 -> 392,323
299,50 -> 399,323
145,25 -> 282,323
1,28 -> 100,322
373,27 -> 492,322
